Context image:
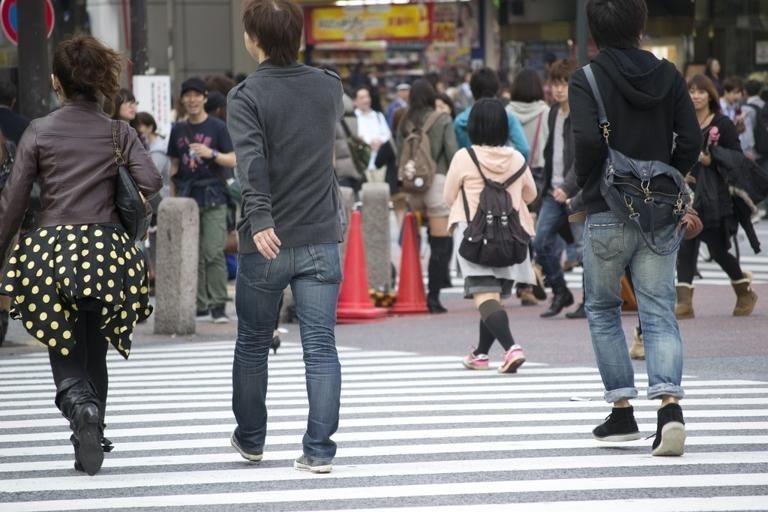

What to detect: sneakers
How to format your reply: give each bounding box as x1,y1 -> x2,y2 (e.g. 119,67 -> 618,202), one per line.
229,432 -> 264,463
293,454 -> 335,475
211,306 -> 229,325
592,405 -> 641,446
505,282 -> 589,319
498,344 -> 525,374
628,326 -> 652,361
460,351 -> 490,371
195,309 -> 209,321
649,402 -> 687,458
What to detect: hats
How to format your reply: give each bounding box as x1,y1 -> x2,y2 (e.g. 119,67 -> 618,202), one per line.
181,77 -> 209,96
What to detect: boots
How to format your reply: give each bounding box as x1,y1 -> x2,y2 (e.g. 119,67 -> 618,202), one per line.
422,232 -> 453,315
53,374 -> 117,476
672,281 -> 697,322
725,271 -> 759,317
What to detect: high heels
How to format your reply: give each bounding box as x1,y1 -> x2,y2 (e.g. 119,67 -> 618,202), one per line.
268,334 -> 282,355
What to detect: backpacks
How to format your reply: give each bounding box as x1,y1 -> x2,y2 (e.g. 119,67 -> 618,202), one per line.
396,107 -> 451,194
453,144 -> 532,265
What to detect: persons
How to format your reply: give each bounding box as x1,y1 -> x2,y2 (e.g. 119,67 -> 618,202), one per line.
0,83 -> 33,342
445,97 -> 543,375
564,0 -> 705,458
332,59 -> 586,318
624,72 -> 768,358
224,1 -> 348,473
111,73 -> 249,322
1,34 -> 162,475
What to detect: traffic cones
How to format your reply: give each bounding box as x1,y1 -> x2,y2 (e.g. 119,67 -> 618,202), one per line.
334,211 -> 390,322
384,212 -> 431,317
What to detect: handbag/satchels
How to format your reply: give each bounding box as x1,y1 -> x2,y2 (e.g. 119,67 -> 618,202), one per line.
597,146 -> 695,234
114,161 -> 155,245
347,134 -> 372,172
745,101 -> 768,156
